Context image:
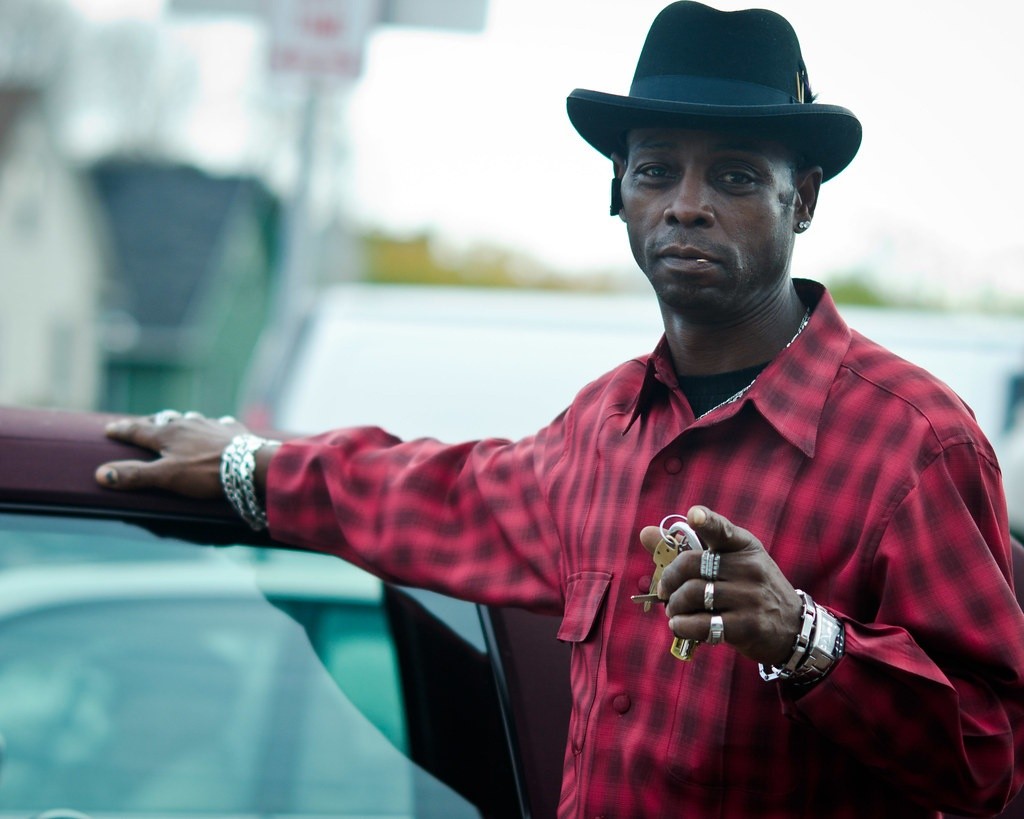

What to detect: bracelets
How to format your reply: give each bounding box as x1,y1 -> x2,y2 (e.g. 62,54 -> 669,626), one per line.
758,589 -> 816,686
219,433 -> 280,532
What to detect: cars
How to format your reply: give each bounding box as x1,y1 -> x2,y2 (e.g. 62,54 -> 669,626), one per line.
5,408 -> 571,819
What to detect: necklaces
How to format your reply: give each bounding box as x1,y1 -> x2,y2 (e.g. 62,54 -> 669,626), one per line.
695,302 -> 813,426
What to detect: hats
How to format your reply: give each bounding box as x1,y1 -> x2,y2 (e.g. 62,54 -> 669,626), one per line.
564,0 -> 865,178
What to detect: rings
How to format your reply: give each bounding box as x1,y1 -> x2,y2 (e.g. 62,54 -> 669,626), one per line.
705,614 -> 725,647
699,547 -> 721,581
703,580 -> 715,612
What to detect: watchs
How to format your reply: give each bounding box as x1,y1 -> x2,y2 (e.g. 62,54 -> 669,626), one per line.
771,600 -> 845,690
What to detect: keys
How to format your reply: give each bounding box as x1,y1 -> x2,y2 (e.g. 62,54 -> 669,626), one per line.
630,513 -> 704,662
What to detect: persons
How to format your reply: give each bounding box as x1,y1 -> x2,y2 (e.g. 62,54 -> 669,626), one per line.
92,0 -> 1023,819
